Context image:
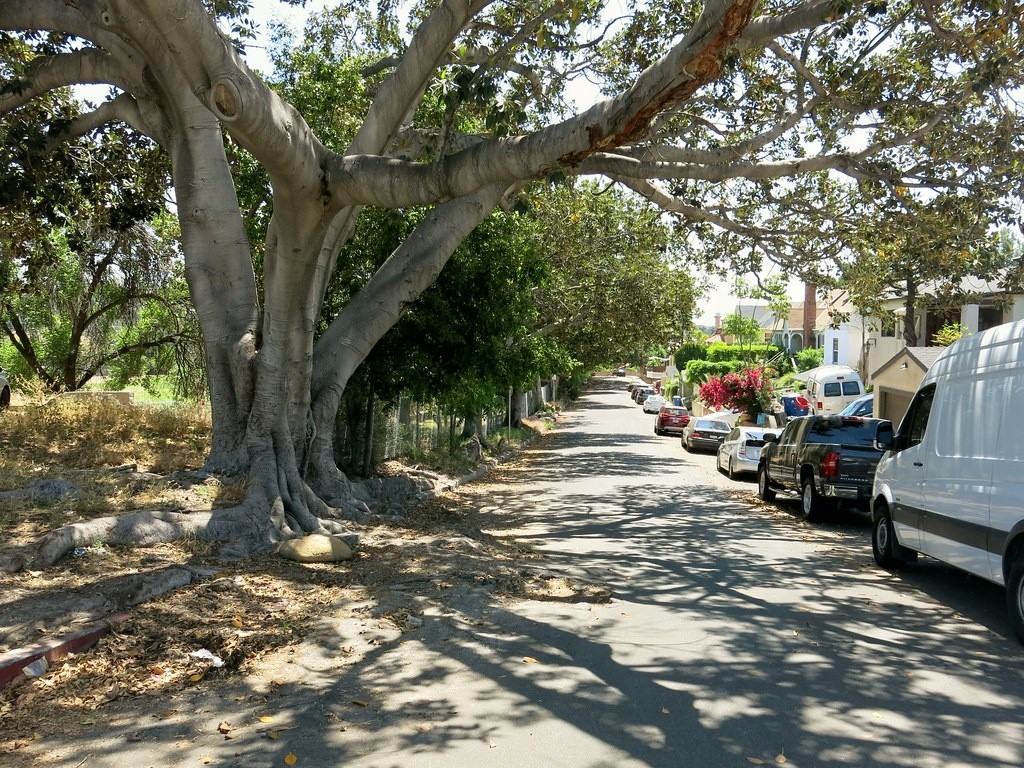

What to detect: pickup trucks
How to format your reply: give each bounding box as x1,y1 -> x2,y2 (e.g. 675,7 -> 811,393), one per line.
758,413 -> 897,522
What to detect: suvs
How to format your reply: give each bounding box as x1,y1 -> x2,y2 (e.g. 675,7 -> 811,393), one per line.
654,405 -> 691,436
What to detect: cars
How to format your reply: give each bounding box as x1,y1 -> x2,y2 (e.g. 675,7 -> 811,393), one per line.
717,426 -> 782,480
839,393 -> 874,417
632,384 -> 654,403
681,417 -> 732,453
643,395 -> 666,413
0,366 -> 11,408
612,369 -> 626,377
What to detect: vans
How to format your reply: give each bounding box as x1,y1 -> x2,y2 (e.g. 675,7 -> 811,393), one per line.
806,366 -> 865,417
870,321 -> 1024,643
